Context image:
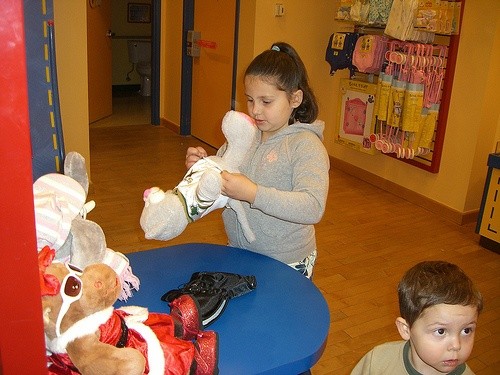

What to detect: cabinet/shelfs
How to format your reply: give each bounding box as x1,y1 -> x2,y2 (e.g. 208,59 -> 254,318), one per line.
324,0 -> 466,174
475,153 -> 500,254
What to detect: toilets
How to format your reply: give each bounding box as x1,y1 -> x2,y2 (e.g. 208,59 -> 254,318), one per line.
128,42 -> 151,96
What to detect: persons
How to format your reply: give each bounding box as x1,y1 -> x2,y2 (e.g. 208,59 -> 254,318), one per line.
184,42 -> 330,279
350,261 -> 483,375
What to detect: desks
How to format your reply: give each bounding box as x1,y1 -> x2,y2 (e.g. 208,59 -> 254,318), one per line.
113,242 -> 330,375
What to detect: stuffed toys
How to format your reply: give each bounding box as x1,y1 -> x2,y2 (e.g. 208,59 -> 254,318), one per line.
32,151 -> 218,375
140,110 -> 257,244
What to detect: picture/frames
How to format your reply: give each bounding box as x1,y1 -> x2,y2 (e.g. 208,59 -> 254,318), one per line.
126,2 -> 154,23
